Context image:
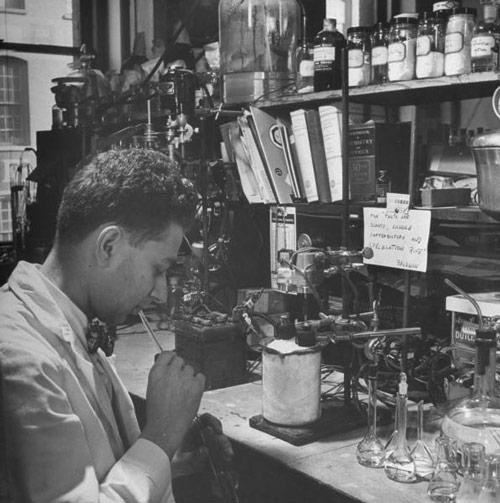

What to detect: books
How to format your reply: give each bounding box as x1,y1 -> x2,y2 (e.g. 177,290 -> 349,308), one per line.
217,101 -> 404,205
269,205 -> 345,295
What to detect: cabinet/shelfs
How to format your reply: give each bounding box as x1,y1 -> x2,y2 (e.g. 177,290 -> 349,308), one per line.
190,69 -> 500,233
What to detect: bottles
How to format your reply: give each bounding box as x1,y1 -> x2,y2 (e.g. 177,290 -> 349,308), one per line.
360,328 -> 500,503
375,169 -> 391,203
294,2 -> 498,92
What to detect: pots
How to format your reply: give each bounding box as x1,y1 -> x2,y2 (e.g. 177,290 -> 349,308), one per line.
473,130 -> 499,212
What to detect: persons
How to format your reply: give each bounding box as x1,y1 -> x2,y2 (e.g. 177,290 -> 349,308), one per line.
0,147 -> 235,503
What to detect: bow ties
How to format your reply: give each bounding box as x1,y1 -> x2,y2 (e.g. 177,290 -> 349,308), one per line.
87,320 -> 117,356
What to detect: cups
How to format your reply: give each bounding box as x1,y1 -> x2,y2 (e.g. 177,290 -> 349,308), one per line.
8,162 -> 33,255
256,339 -> 323,425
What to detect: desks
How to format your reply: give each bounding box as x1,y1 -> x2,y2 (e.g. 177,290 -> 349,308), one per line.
99,328 -> 500,503
